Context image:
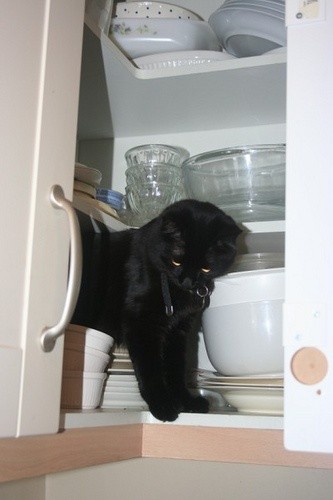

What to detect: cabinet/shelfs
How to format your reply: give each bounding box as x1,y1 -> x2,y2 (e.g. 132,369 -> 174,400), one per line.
0,0 -> 333,485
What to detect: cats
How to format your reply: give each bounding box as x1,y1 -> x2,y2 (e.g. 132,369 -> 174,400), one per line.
67,198 -> 244,423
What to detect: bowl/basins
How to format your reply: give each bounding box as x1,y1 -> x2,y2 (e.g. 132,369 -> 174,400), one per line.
60,370 -> 107,410
124,144 -> 185,167
65,322 -> 114,353
124,182 -> 182,227
225,253 -> 283,272
125,162 -> 182,187
62,342 -> 110,373
181,143 -> 285,221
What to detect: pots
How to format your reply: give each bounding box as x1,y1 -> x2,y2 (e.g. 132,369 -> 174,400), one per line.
200,268 -> 283,376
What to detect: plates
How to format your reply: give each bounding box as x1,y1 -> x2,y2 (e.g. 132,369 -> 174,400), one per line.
208,0 -> 285,59
73,162 -> 124,221
104,17 -> 236,69
99,342 -> 149,409
198,369 -> 283,415
